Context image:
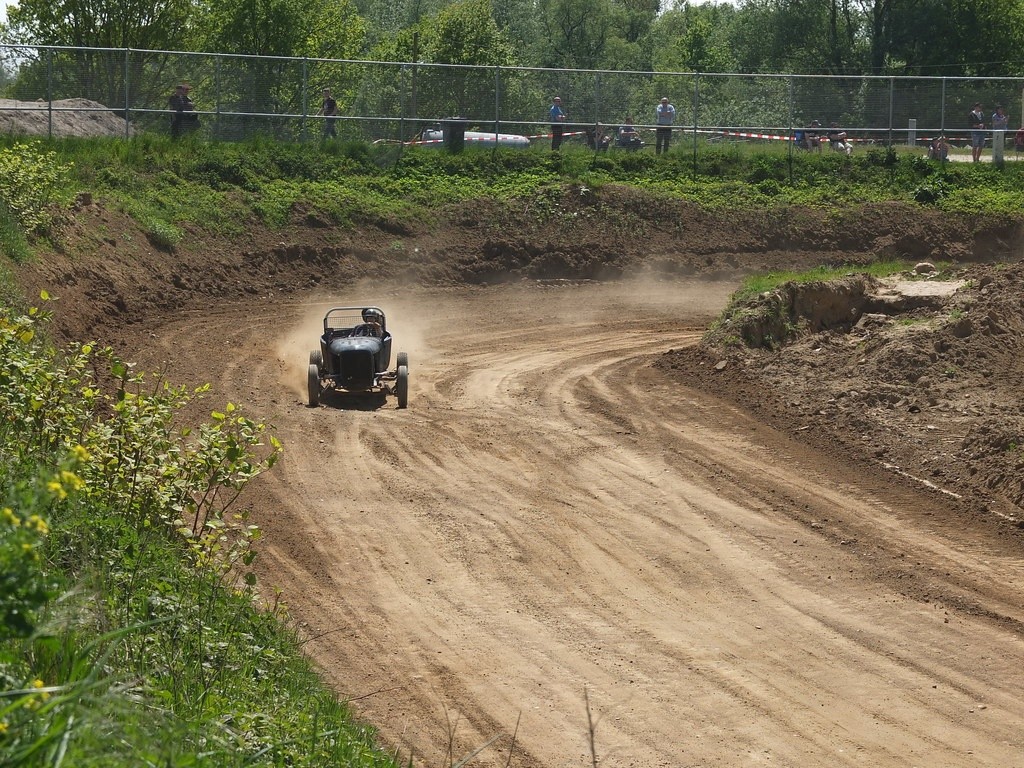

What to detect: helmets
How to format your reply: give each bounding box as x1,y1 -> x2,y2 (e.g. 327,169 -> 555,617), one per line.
364,309 -> 377,321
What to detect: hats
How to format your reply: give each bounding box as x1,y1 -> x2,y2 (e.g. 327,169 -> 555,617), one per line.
812,120 -> 822,126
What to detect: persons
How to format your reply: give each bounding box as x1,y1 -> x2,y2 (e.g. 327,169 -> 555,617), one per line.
655,97 -> 675,154
928,136 -> 949,162
827,122 -> 853,156
619,117 -> 641,151
1016,127 -> 1024,152
992,105 -> 1010,163
589,121 -> 610,153
968,101 -> 986,163
794,120 -> 822,154
348,309 -> 383,338
316,88 -> 338,144
549,96 -> 565,151
169,85 -> 194,142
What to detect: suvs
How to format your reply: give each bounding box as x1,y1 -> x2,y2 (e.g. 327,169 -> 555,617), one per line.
308,307 -> 410,409
